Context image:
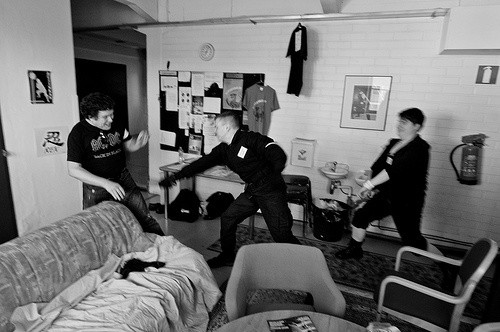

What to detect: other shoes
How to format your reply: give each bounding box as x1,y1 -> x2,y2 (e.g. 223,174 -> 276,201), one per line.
206,252 -> 234,269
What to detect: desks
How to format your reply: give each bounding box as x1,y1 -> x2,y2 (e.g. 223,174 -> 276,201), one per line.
159,157 -> 254,239
212,310 -> 370,332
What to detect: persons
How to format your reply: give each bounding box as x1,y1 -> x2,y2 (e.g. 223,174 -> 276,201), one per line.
337,108 -> 446,263
158,110 -> 300,269
66,92 -> 165,237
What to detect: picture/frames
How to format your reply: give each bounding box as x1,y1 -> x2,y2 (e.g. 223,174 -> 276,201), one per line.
291,138 -> 315,167
339,75 -> 393,131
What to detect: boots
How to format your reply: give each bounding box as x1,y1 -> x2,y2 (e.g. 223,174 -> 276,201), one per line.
432,254 -> 459,291
334,236 -> 366,260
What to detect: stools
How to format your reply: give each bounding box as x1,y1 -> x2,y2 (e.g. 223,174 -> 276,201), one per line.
283,176 -> 313,238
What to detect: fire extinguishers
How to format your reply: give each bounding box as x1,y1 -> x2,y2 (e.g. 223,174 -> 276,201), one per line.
448,133 -> 485,186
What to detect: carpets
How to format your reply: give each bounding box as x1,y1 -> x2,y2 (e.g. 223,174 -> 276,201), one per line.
206,224 -> 493,320
207,276 -> 474,332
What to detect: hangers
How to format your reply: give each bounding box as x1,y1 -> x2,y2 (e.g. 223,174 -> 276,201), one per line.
254,73 -> 267,86
298,14 -> 302,28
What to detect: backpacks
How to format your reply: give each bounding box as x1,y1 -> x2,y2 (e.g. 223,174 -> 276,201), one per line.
167,187 -> 205,222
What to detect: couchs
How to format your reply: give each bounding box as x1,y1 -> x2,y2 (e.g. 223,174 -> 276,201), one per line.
0,201 -> 222,332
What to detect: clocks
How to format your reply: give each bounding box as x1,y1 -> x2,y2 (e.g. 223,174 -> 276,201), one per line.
199,43 -> 215,62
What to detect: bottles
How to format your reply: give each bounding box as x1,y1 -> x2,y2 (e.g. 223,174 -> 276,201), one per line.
179,147 -> 184,163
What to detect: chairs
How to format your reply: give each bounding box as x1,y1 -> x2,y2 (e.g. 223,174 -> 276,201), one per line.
225,243 -> 346,322
373,236 -> 498,332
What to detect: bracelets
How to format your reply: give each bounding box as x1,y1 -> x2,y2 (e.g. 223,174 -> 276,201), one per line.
363,179 -> 375,190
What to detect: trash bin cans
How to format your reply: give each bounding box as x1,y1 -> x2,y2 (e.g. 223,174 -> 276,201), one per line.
311,198 -> 352,241
